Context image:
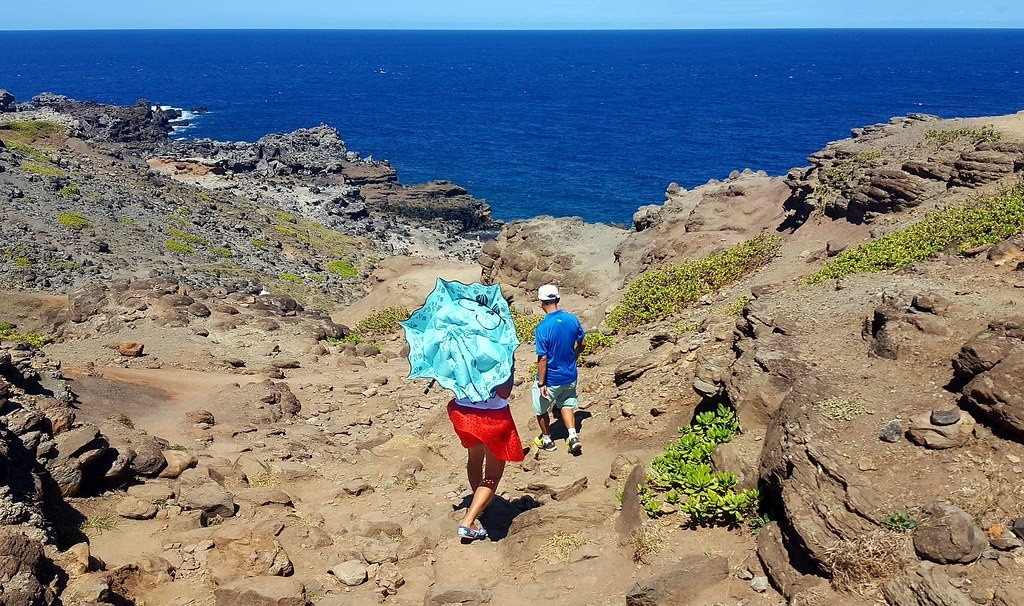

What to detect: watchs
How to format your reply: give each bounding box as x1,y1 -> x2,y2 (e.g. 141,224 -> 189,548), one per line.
538,382 -> 546,388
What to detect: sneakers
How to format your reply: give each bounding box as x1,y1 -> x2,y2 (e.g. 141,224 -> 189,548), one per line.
457,525 -> 488,540
565,437 -> 582,453
533,436 -> 557,451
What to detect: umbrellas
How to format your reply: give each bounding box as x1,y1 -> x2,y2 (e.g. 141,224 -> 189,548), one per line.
398,276 -> 522,405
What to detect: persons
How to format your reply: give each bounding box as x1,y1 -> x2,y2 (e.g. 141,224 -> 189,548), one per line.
530,285 -> 587,452
447,358 -> 524,539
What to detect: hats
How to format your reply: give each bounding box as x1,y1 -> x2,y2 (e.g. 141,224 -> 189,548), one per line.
538,284 -> 560,300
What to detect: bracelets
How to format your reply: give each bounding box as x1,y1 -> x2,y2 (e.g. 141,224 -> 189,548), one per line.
511,367 -> 516,371
